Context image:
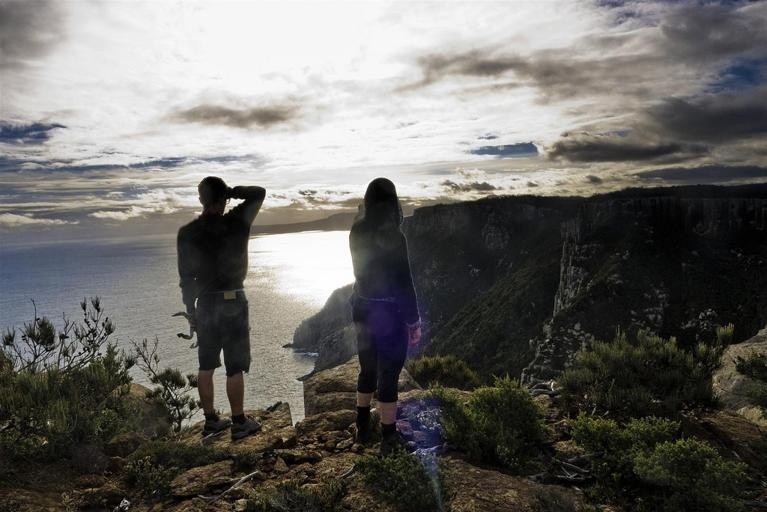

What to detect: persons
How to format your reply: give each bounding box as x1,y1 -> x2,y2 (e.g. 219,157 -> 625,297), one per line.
349,179 -> 423,445
178,176 -> 266,440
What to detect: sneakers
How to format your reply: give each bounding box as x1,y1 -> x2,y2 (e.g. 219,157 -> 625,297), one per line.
355,428 -> 382,445
230,413 -> 262,440
379,431 -> 407,456
203,417 -> 232,433
